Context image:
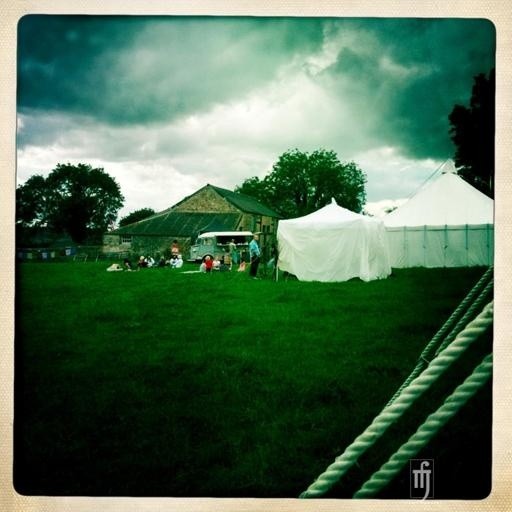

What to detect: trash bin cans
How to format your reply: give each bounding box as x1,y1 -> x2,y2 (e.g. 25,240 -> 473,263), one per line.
65,247 -> 76,256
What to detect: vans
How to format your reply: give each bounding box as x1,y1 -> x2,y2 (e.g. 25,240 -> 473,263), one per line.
190,232 -> 254,264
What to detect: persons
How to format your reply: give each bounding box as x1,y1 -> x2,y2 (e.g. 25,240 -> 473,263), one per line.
170,239 -> 179,255
268,243 -> 277,266
235,257 -> 246,272
248,235 -> 261,279
124,255 -> 184,270
228,238 -> 237,264
200,255 -> 233,272
